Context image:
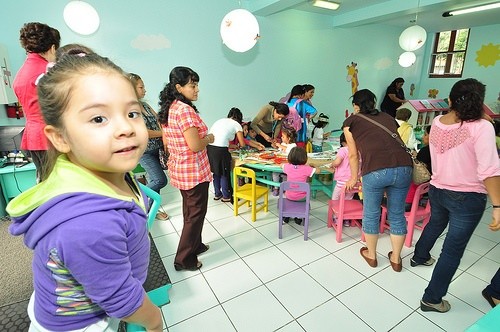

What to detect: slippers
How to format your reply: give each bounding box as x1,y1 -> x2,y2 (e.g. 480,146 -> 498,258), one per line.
214,195 -> 223,200
221,197 -> 240,202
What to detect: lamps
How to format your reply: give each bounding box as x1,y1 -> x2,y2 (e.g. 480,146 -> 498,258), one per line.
442,1 -> 500,16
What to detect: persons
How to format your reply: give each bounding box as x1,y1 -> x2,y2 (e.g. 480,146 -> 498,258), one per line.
206,107 -> 245,202
413,125 -> 432,198
380,78 -> 406,118
14,22 -> 61,183
411,78 -> 500,313
395,108 -> 417,149
279,147 -> 320,224
490,119 -> 500,157
272,127 -> 298,196
250,101 -> 290,147
156,66 -> 214,271
329,132 -> 362,226
127,73 -> 169,221
228,121 -> 265,185
286,84 -> 331,140
404,182 -> 424,212
4,44 -> 163,332
342,89 -> 414,272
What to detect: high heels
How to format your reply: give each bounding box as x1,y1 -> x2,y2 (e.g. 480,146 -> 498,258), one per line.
420,300 -> 451,313
410,257 -> 435,267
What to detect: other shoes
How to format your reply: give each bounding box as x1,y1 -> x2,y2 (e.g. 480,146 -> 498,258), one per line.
294,217 -> 302,225
360,247 -> 377,267
175,262 -> 202,271
482,288 -> 497,309
273,191 -> 278,196
283,217 -> 289,223
344,221 -> 349,226
334,219 -> 337,225
196,245 -> 209,255
388,251 -> 402,272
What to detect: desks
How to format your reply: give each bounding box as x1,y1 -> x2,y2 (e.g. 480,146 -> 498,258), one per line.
230,132 -> 340,198
0,155 -> 37,210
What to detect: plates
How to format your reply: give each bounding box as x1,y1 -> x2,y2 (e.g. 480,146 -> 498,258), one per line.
275,158 -> 286,164
259,152 -> 276,159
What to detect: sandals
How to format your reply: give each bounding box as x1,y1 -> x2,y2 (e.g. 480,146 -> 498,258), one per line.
155,210 -> 168,221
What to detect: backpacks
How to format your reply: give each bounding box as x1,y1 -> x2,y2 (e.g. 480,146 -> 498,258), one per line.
283,98 -> 304,132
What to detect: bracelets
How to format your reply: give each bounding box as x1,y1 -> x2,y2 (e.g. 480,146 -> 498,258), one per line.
493,205 -> 500,208
205,136 -> 210,141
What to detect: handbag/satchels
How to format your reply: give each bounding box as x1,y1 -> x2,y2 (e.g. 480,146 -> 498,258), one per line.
412,158 -> 431,185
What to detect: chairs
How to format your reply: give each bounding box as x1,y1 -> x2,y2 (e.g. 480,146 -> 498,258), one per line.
380,183 -> 430,247
278,182 -> 309,241
233,167 -> 269,222
328,177 -> 365,243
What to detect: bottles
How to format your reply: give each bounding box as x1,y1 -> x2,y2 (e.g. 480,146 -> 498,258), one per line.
306,141 -> 312,153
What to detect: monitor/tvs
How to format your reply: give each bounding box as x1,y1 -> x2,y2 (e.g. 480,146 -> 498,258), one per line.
0,126 -> 26,164
